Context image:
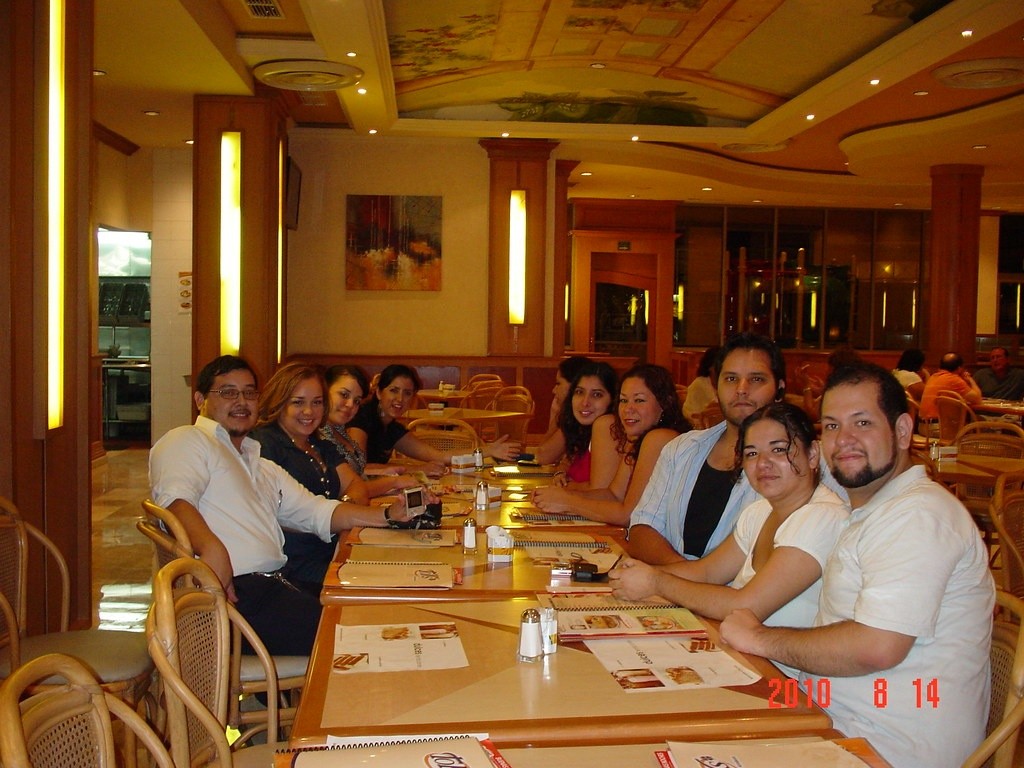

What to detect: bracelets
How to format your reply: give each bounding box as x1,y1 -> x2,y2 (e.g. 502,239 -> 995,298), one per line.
385,505 -> 399,527
803,388 -> 812,392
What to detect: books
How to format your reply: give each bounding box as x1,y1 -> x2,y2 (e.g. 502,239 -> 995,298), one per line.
323,466 -> 703,642
666,736 -> 873,768
273,735 -> 496,768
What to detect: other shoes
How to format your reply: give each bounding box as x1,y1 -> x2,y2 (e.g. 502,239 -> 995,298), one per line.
238,694 -> 286,746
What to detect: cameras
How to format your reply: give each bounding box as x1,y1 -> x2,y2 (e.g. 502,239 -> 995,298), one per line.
403,485 -> 424,517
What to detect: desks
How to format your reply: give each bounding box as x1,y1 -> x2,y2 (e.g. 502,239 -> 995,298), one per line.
967,397 -> 1023,421
280,446 -> 894,768
398,405 -> 527,431
99,364 -> 152,441
414,389 -> 504,406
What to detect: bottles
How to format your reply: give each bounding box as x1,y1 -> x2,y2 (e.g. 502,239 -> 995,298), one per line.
476,481 -> 489,511
439,381 -> 444,393
928,441 -> 939,462
474,448 -> 485,472
516,610 -> 546,662
462,518 -> 478,555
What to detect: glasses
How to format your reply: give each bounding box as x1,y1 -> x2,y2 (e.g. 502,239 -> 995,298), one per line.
209,387 -> 259,401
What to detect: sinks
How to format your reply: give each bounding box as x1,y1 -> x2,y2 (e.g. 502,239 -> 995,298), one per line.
102,358 -> 151,367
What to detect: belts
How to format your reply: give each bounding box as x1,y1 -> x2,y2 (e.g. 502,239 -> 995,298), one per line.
919,419 -> 938,423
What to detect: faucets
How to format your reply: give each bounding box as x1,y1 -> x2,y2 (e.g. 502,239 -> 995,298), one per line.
107,313 -> 116,345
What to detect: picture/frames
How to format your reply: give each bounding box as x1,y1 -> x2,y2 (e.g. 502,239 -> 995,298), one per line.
285,156 -> 302,231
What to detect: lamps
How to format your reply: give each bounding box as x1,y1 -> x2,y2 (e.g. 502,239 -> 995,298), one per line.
508,188 -> 526,326
219,128 -> 243,359
32,0 -> 65,441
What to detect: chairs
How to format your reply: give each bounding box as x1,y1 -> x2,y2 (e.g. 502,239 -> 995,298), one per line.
390,371 -> 536,460
0,495 -> 311,768
901,389 -> 1024,768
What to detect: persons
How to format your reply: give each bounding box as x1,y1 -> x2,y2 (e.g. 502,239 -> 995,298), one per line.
719,362 -> 996,768
247,356 -> 520,566
539,336 -> 1024,679
147,355 -> 440,707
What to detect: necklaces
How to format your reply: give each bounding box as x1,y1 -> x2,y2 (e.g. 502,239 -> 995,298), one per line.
289,436 -> 331,498
